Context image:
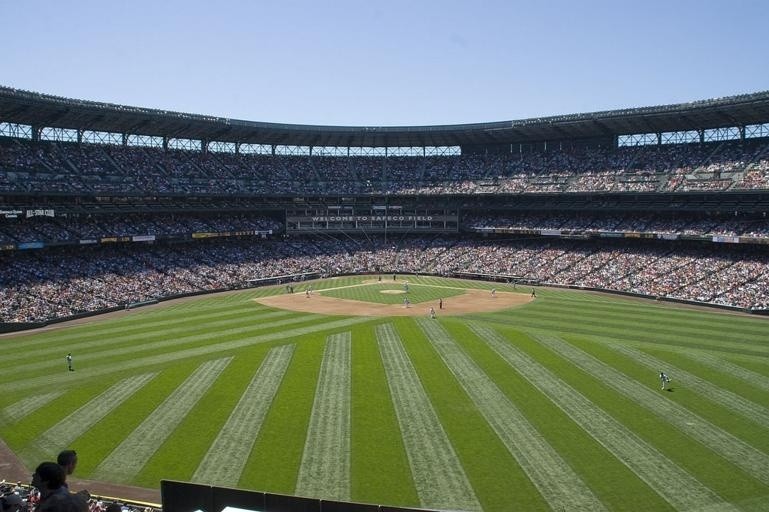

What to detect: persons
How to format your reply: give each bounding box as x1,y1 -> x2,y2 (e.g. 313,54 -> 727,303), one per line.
660,372 -> 671,390
66,351 -> 73,370
1,141 -> 769,319
57,450 -> 78,487
31,462 -> 67,512
0,479 -> 159,512
